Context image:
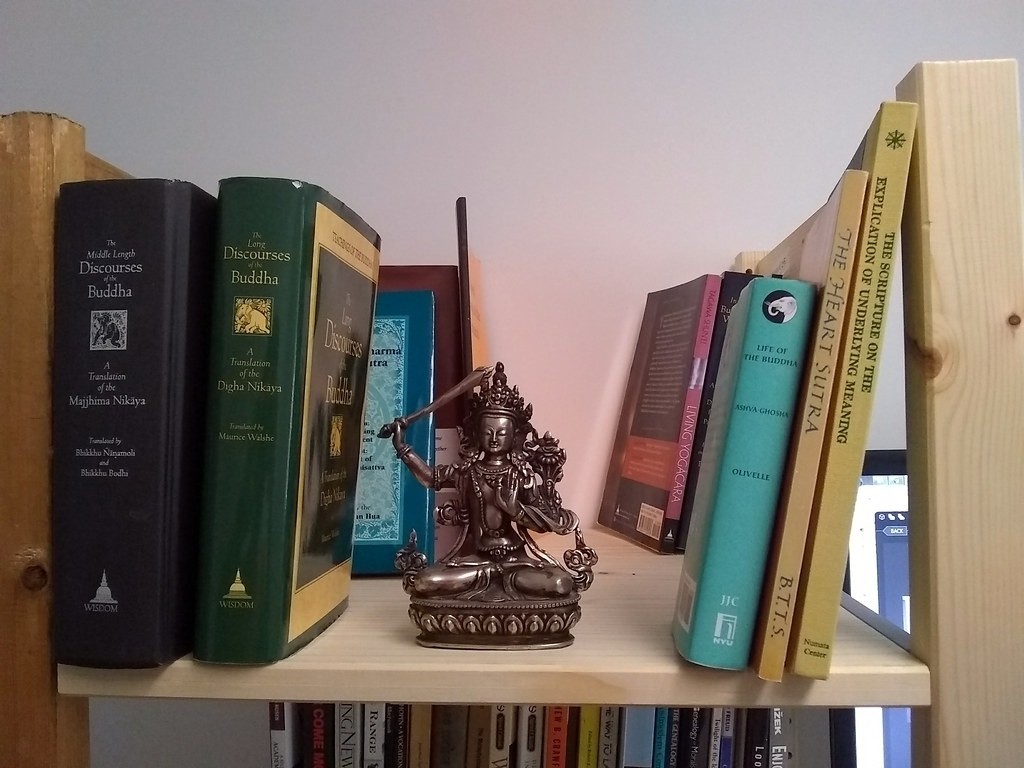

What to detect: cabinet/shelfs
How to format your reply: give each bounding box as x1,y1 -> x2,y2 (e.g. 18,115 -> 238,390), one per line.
0,57 -> 1024,768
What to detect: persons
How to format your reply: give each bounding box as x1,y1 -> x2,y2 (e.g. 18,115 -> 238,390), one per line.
397,362 -> 578,602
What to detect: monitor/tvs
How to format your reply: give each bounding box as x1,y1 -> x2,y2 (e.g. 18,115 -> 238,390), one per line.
829,448 -> 912,768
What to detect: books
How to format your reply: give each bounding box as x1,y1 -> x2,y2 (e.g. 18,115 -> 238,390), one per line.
47,175 -> 467,670
268,697 -> 797,767
596,99 -> 922,683
